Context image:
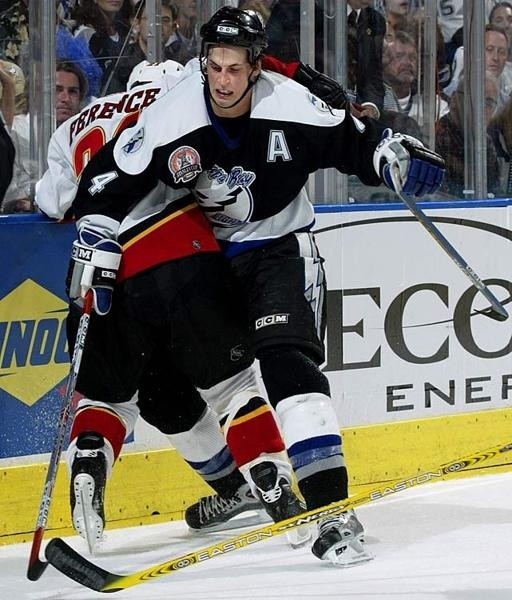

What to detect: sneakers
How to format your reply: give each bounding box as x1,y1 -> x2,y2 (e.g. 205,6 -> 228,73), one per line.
185,482 -> 266,530
311,508 -> 366,560
250,461 -> 308,524
69,430 -> 107,545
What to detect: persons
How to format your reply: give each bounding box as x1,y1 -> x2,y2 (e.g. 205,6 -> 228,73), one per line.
34,57 -> 305,543
429,70 -> 502,198
64,5 -> 447,561
1,1 -> 197,215
436,0 -> 511,163
262,0 -> 423,128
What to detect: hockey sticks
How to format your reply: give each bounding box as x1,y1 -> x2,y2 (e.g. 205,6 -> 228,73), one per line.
27,291 -> 94,581
45,443 -> 512,594
391,159 -> 509,322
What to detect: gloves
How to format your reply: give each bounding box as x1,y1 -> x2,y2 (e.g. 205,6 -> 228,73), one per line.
291,61 -> 351,115
373,133 -> 446,197
65,214 -> 124,316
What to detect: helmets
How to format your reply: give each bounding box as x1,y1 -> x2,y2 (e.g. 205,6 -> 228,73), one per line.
125,59 -> 185,92
199,6 -> 269,50
